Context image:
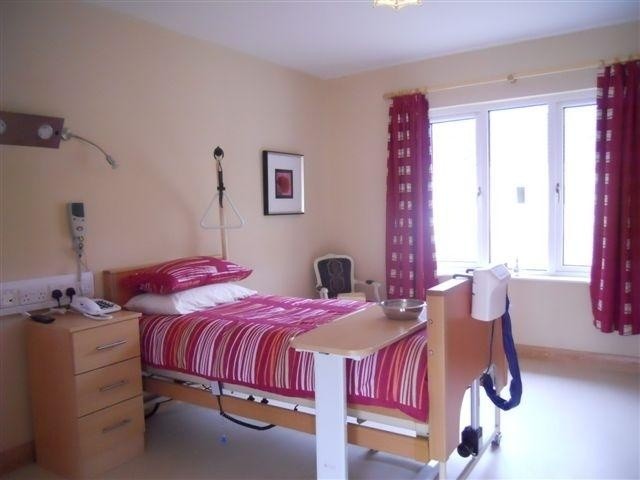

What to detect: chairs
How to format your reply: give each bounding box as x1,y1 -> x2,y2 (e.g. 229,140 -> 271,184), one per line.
312,252 -> 381,303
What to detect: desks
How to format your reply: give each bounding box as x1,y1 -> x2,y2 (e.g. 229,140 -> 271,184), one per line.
287,305 -> 428,480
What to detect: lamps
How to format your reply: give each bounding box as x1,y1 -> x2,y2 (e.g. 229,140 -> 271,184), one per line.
57,128 -> 119,170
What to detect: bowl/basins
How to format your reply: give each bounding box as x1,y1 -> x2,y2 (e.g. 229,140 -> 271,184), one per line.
380,298 -> 425,319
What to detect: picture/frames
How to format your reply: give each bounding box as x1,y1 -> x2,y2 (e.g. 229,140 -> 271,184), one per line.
262,149 -> 306,215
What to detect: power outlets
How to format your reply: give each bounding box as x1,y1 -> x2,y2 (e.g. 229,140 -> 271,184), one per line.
0,271 -> 95,316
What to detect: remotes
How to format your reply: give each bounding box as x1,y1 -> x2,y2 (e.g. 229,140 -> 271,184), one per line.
30,314 -> 56,324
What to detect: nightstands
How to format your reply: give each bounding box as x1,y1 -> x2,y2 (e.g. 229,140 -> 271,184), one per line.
24,308 -> 146,479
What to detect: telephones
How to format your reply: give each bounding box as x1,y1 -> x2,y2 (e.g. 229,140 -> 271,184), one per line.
70,296 -> 121,316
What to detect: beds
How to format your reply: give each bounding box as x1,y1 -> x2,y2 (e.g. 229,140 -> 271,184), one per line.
102,268 -> 509,480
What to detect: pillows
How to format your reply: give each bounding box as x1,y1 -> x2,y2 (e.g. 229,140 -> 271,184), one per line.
121,254 -> 259,315
